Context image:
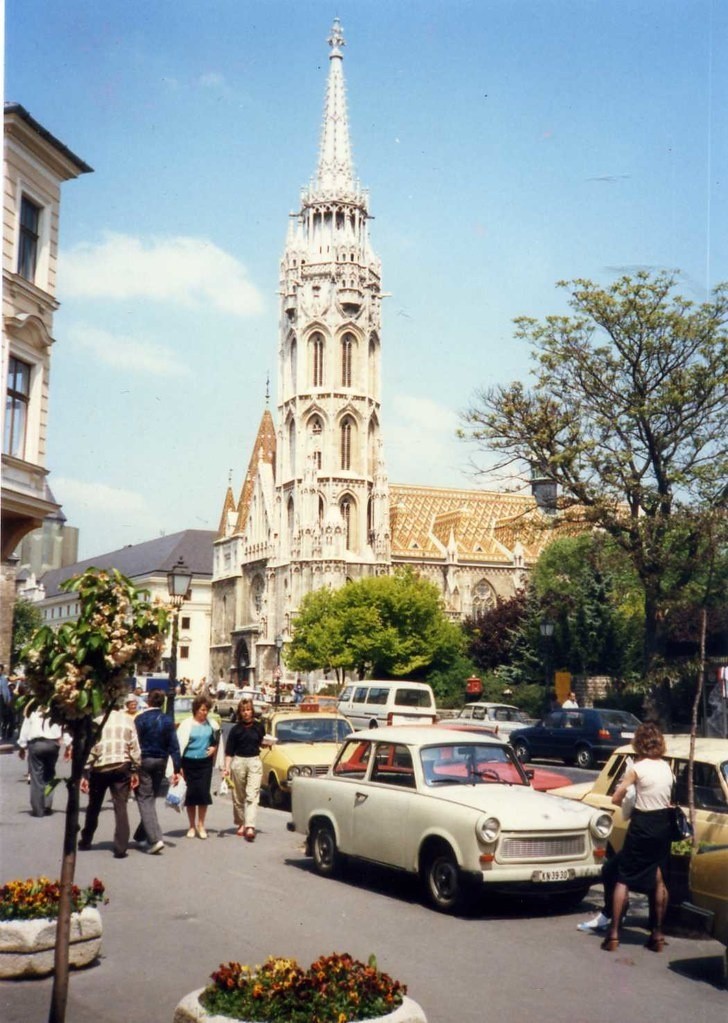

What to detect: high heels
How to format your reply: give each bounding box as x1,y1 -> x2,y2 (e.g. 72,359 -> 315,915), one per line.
600,937 -> 619,951
644,934 -> 665,952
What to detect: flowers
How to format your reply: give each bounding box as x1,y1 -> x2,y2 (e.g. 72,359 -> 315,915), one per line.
0,875 -> 110,922
198,951 -> 408,1023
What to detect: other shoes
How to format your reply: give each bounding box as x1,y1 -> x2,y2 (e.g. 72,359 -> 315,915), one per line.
146,840 -> 164,854
186,825 -> 207,839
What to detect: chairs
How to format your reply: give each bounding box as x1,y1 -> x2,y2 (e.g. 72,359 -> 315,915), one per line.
420,760 -> 442,778
678,765 -> 721,806
277,730 -> 293,741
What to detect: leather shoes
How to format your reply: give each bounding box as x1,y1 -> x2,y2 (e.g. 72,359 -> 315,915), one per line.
237,824 -> 256,839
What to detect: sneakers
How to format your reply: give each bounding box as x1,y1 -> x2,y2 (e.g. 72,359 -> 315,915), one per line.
576,912 -> 609,933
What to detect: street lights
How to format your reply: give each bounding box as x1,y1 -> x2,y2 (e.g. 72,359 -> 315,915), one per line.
274,634 -> 284,707
539,616 -> 554,711
166,556 -> 193,721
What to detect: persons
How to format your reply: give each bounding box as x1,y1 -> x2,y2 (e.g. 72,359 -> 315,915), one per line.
79,693 -> 142,858
17,698 -> 73,817
134,689 -> 182,854
175,678 -> 306,708
577,724 -> 677,950
544,691 -> 579,727
222,699 -> 272,838
0,662 -> 36,741
165,696 -> 220,839
122,687 -> 148,803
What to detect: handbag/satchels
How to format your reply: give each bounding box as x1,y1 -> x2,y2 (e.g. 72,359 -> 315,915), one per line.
165,773 -> 188,814
219,776 -> 234,795
672,780 -> 694,842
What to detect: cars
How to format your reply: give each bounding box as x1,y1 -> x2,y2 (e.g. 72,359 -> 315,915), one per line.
162,695 -> 221,729
438,702 -> 531,744
286,726 -> 615,913
544,738 -> 728,920
258,711 -> 370,809
336,724 -> 573,791
213,689 -> 272,723
509,708 -> 643,770
301,695 -> 338,713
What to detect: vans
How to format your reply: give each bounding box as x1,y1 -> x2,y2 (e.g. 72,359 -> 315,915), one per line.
336,681 -> 439,731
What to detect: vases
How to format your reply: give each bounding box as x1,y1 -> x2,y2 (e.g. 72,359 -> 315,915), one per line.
0,907 -> 103,977
175,986 -> 426,1023
